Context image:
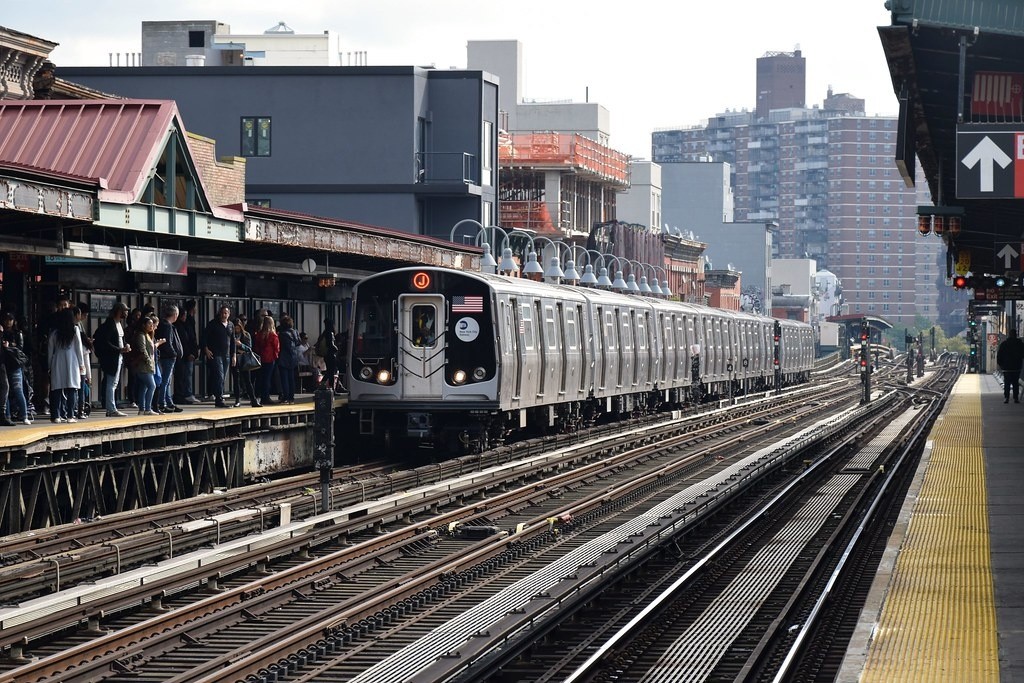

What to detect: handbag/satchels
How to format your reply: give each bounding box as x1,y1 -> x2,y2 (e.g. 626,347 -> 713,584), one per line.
238,347 -> 262,371
0,346 -> 29,371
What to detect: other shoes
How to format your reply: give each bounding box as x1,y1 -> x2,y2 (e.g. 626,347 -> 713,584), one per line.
0,418 -> 16,426
78,410 -> 87,419
234,402 -> 240,407
67,417 -> 77,423
251,399 -> 264,407
24,420 -> 31,425
335,393 -> 340,395
54,418 -> 61,423
167,404 -> 183,412
110,410 -> 128,417
260,398 -> 276,404
289,400 -> 295,405
60,416 -> 65,421
182,395 -> 201,404
279,400 -> 289,405
158,406 -> 173,412
214,403 -> 230,408
143,409 -> 159,415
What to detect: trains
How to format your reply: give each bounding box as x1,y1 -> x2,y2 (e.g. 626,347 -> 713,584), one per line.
344,266 -> 816,455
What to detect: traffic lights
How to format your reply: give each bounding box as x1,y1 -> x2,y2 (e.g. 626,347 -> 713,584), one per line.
860,353 -> 867,374
861,321 -> 868,340
774,347 -> 779,365
774,323 -> 780,341
956,276 -> 967,288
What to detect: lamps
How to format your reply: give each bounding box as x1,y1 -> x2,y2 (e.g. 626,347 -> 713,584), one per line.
633,263 -> 663,293
644,266 -> 673,294
300,254 -> 318,273
607,256 -> 641,291
501,231 -> 544,272
561,245 -> 598,283
948,216 -> 961,238
524,237 -> 565,277
918,215 -> 932,237
595,254 -> 629,289
541,241 -> 581,279
619,260 -> 652,292
934,216 -> 947,236
475,226 -> 519,270
579,250 -> 613,285
450,219 -> 497,266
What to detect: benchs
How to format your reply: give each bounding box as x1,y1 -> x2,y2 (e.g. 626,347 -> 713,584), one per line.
297,362 -> 314,393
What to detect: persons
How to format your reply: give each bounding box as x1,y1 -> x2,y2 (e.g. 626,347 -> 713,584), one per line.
997,329 -> 1024,403
0,295 -> 351,427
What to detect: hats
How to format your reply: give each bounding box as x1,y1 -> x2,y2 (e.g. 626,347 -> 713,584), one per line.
5,313 -> 14,319
112,303 -> 130,311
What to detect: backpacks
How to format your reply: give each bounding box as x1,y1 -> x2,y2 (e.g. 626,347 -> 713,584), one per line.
313,332 -> 328,358
